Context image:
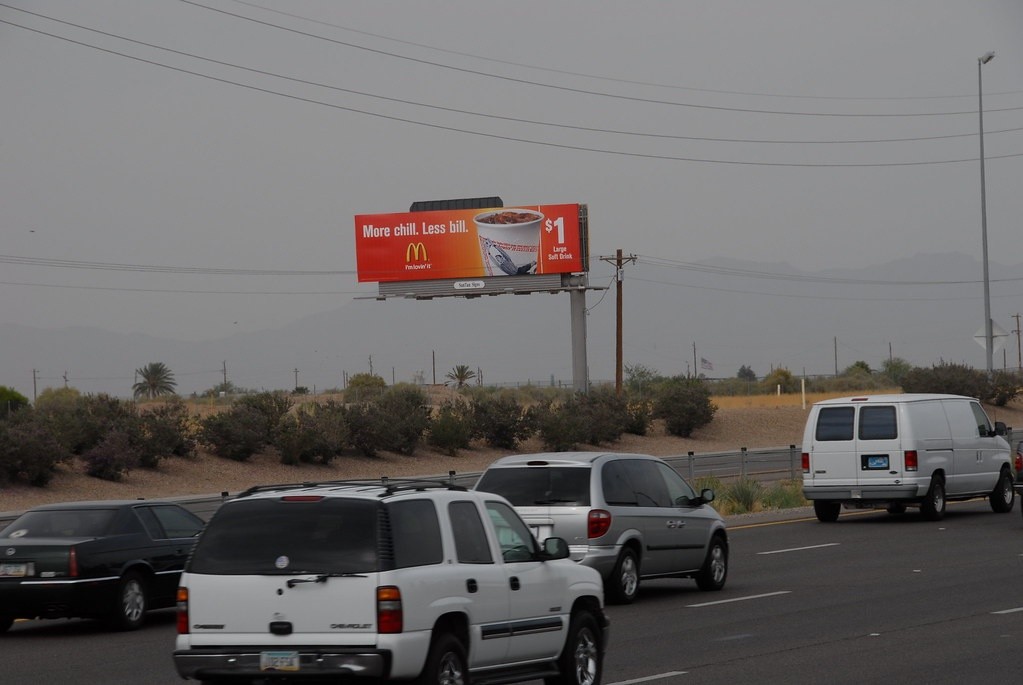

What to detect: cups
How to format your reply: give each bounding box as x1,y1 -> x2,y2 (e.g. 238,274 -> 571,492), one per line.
473,209 -> 544,276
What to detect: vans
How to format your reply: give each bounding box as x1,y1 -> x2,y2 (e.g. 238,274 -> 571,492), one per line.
800,392 -> 1018,521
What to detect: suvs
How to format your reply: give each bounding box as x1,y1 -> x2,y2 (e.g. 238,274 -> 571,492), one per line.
167,471 -> 611,685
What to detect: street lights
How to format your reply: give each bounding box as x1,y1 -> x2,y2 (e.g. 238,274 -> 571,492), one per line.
977,50 -> 999,404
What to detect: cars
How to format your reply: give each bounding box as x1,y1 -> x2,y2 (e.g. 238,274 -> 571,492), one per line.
468,452 -> 731,607
0,499 -> 210,636
